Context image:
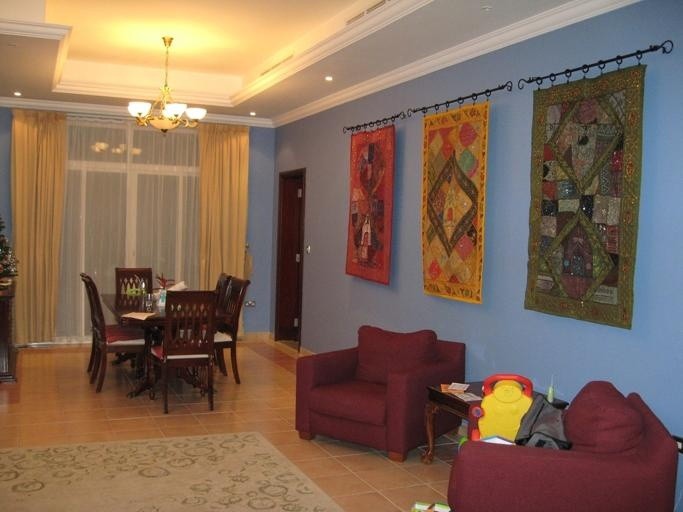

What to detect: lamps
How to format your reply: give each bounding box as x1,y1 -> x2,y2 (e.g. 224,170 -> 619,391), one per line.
127,31 -> 205,136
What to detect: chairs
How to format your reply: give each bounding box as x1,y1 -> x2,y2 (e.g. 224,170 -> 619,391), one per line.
76,267 -> 255,416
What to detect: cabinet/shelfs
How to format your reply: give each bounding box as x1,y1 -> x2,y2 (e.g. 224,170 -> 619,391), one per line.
1,270 -> 19,384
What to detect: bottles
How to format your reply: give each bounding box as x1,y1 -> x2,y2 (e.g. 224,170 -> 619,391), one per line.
547,385 -> 553,403
140,281 -> 162,311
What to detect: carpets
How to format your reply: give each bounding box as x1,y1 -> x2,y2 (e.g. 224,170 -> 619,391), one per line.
1,429 -> 344,511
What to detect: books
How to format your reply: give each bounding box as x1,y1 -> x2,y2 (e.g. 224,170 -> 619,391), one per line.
457,425 -> 468,436
459,418 -> 469,426
409,501 -> 431,511
426,501 -> 450,512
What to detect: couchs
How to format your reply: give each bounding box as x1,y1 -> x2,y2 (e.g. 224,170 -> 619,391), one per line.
444,378 -> 679,512
292,322 -> 472,464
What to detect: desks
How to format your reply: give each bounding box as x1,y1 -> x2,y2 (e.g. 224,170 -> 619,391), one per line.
417,379 -> 570,463
